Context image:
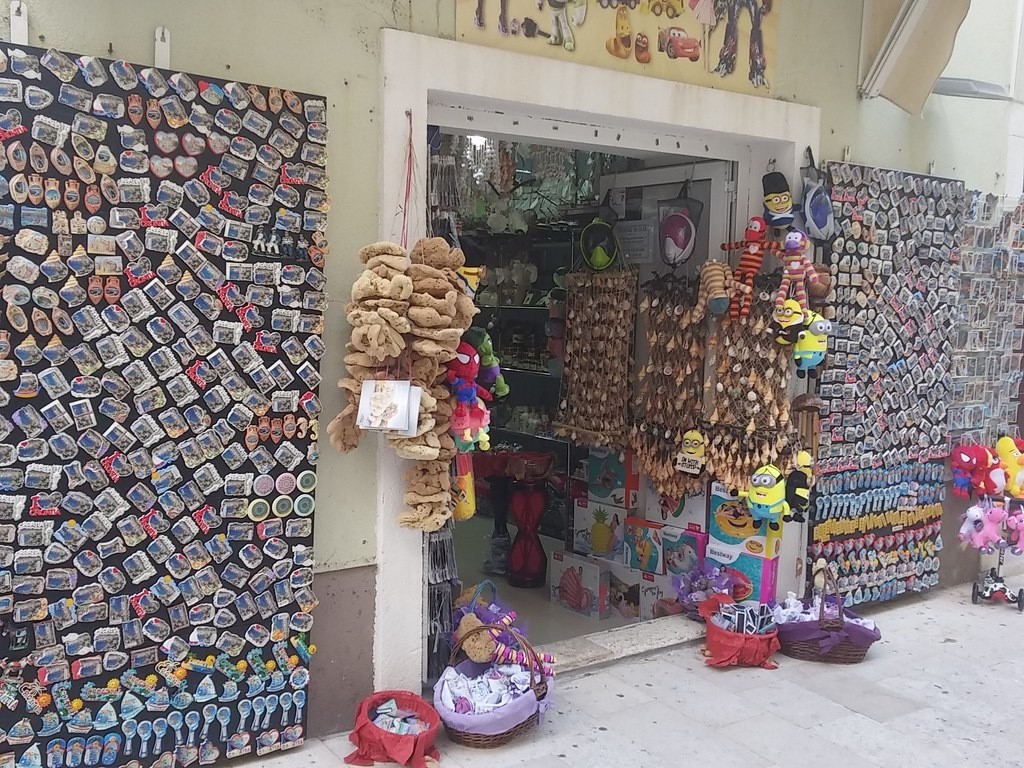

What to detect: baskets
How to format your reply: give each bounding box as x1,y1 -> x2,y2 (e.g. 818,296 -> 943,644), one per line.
678,570 -> 722,622
775,567 -> 881,664
451,579 -> 520,667
433,623 -> 553,749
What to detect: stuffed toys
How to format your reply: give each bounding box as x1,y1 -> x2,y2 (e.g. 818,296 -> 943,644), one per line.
786,451 -> 816,521
691,171 -> 835,378
448,235 -> 509,456
454,585 -> 556,677
729,464 -> 791,530
672,430 -> 707,479
949,435 -> 1024,557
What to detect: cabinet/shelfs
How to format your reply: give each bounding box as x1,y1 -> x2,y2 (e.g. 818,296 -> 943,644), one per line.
459,224 -> 583,540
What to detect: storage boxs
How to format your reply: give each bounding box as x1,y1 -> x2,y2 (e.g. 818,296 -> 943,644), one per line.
551,446 -> 784,622
